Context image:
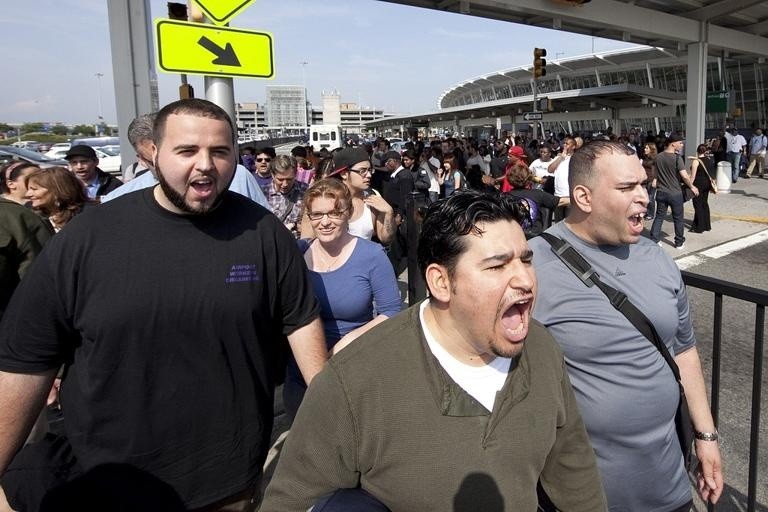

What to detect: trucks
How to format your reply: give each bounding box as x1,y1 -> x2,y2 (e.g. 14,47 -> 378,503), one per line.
309,123 -> 342,155
660,127 -> 763,169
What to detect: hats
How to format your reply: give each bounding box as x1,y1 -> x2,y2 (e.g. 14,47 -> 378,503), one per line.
382,150 -> 403,163
507,146 -> 528,158
323,148 -> 371,179
668,133 -> 686,142
63,144 -> 97,160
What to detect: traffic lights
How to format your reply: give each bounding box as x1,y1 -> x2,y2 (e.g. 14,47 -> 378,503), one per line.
533,47 -> 547,80
177,83 -> 194,100
538,98 -> 553,112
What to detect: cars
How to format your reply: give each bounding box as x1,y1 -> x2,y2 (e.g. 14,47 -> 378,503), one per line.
342,129 -> 463,158
237,130 -> 309,145
0,134 -> 122,188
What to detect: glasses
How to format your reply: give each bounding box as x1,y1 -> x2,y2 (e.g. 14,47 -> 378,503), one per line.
347,167 -> 376,177
307,206 -> 353,221
255,157 -> 272,162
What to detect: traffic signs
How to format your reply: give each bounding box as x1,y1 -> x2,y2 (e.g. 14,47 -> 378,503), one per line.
523,111 -> 543,121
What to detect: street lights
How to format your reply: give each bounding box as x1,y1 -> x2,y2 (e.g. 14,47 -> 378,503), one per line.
94,73 -> 106,118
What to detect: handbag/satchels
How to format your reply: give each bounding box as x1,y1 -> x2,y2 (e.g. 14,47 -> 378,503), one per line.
709,180 -> 719,194
